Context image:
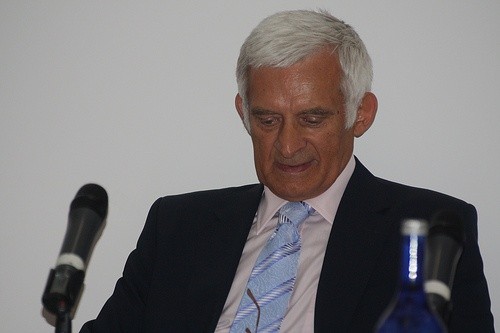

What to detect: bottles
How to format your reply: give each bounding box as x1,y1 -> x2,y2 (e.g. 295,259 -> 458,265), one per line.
372,218 -> 446,333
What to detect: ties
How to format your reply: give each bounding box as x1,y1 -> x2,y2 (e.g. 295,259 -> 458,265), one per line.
230,202 -> 316,333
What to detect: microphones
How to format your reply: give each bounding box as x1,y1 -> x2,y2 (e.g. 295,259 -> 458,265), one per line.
43,184 -> 108,315
422,208 -> 465,311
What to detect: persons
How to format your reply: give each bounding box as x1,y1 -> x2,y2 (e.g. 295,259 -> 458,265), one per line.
80,9 -> 495,333
